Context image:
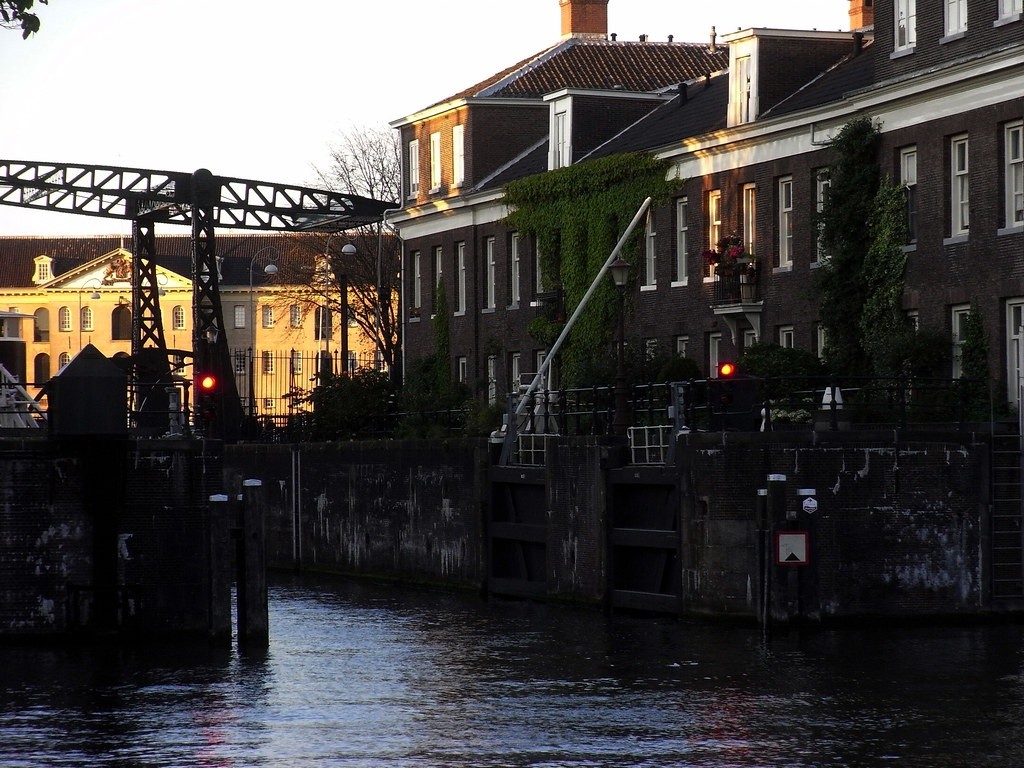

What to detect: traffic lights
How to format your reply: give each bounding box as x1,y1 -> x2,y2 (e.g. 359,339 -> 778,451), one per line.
200,374 -> 218,421
718,362 -> 736,413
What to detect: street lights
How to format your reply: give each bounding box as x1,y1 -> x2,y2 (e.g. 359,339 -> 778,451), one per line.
609,254 -> 630,437
249,245 -> 280,441
325,228 -> 360,380
